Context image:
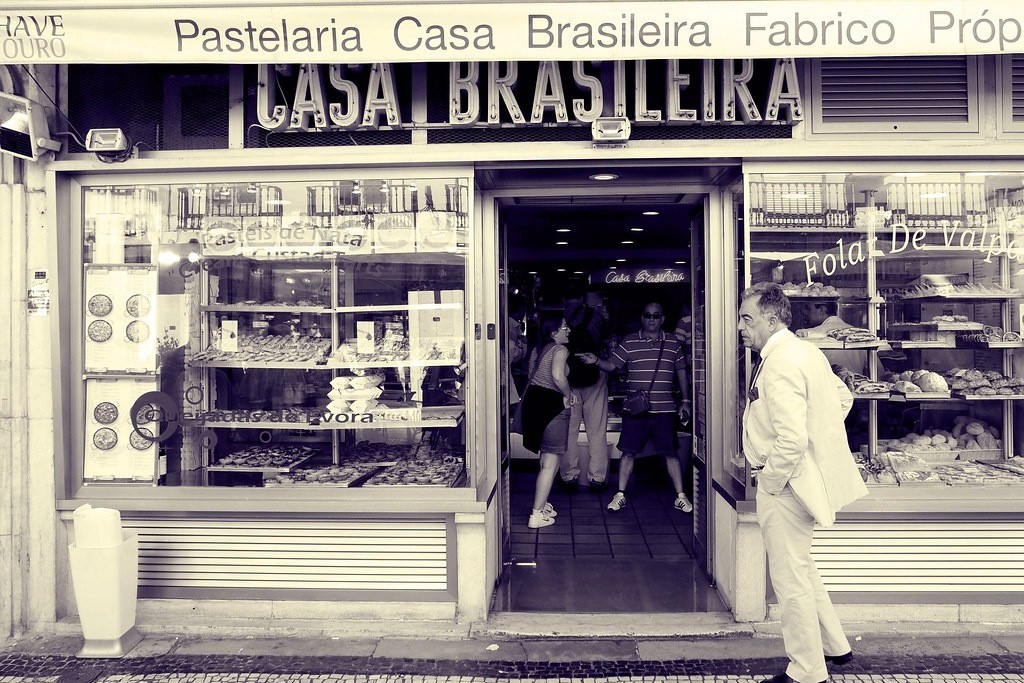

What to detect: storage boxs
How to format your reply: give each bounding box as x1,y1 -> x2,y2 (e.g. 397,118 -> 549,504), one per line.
957,447 -> 1003,462
876,439 -> 959,462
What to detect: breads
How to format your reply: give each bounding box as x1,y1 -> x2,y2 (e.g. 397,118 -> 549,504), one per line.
779,281 -> 1024,484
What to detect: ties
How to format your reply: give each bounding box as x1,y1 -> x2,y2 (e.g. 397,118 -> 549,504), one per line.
749,355 -> 762,391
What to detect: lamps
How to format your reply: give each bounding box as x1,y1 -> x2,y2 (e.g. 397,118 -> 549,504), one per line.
0,90 -> 63,163
591,116 -> 633,149
85,128 -> 128,152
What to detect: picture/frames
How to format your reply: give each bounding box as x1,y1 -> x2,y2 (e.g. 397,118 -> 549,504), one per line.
83,376 -> 159,487
82,263 -> 159,377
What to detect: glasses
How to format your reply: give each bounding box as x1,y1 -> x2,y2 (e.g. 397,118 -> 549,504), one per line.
643,312 -> 661,319
560,326 -> 568,331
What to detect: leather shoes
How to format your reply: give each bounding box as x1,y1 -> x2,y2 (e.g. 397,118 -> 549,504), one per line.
824,651 -> 854,665
759,674 -> 827,683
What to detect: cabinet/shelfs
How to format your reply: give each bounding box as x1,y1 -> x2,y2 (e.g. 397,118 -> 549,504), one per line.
197,242 -> 470,488
749,225 -> 1023,488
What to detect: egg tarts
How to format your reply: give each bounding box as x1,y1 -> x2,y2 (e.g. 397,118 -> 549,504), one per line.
219,441 -> 464,487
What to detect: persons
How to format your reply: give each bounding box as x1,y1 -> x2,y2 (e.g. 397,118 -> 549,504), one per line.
585,292 -> 615,356
673,298 -> 693,395
575,303 -> 693,513
739,282 -> 862,682
497,312 -> 523,418
525,315 -> 577,528
556,282 -> 613,490
797,298 -> 890,453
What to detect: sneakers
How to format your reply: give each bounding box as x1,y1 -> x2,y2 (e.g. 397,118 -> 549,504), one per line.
528,502 -> 557,528
673,493 -> 694,513
606,493 -> 626,512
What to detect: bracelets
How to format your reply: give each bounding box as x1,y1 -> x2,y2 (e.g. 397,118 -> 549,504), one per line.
594,357 -> 600,365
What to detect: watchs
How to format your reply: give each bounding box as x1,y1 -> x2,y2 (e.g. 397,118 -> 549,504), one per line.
681,399 -> 690,403
